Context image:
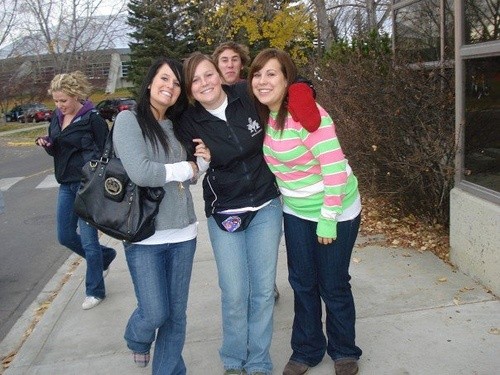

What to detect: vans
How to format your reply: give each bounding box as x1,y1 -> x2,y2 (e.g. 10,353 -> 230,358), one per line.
5,103 -> 47,122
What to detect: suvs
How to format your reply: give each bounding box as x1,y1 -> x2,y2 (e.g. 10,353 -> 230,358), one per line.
95,97 -> 138,122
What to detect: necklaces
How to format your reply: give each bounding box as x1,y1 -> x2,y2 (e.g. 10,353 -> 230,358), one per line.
163,145 -> 186,193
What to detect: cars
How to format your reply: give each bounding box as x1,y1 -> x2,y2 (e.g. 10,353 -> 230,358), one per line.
18,108 -> 53,123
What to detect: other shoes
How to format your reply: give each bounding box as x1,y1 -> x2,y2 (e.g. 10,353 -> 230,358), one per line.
103,252 -> 118,279
282,360 -> 308,374
83,295 -> 105,310
224,368 -> 246,375
334,357 -> 359,375
132,350 -> 151,368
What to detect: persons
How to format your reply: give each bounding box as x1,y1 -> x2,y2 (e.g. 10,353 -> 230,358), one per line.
110,56 -> 211,375
33,73 -> 117,310
213,42 -> 246,85
247,48 -> 365,375
172,51 -> 322,375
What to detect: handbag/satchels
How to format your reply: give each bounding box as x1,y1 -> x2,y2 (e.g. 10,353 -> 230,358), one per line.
212,211 -> 256,232
73,111 -> 166,243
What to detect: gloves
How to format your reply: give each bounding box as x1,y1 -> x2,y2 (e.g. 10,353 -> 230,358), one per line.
287,82 -> 322,133
